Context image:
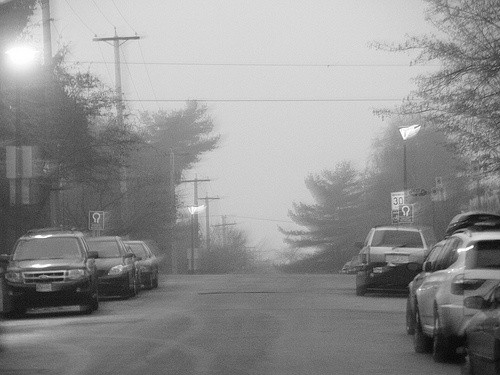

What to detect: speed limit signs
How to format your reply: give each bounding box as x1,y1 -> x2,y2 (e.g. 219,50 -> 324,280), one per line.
391,192 -> 404,210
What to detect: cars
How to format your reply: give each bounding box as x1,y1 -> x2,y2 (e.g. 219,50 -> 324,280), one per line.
339,255 -> 359,274
124,241 -> 159,289
462,283 -> 500,375
87,237 -> 137,298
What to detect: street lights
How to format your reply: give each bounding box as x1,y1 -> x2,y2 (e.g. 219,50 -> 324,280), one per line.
398,126 -> 411,205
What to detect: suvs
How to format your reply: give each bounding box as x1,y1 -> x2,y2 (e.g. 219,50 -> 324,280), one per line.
1,226 -> 97,314
404,211 -> 500,361
354,225 -> 429,296
